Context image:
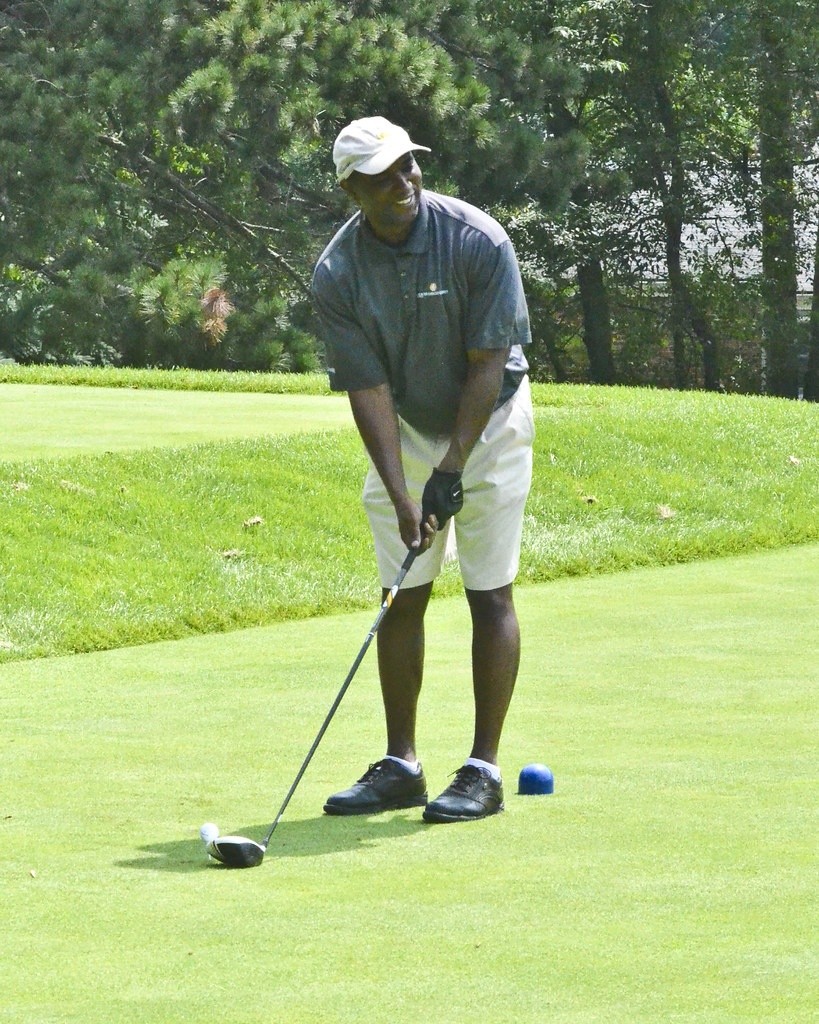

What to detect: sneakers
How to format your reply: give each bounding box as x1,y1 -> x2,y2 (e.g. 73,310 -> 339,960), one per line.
323,759 -> 427,812
424,766 -> 506,821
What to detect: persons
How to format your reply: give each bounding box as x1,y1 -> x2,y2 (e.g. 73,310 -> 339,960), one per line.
309,117 -> 538,823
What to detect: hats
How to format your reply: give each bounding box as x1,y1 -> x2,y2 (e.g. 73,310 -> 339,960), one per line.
334,116 -> 434,185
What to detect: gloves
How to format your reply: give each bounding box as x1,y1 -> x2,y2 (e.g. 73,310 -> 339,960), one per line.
420,468 -> 465,530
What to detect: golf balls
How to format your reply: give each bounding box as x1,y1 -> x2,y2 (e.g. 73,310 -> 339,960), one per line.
199,820 -> 220,842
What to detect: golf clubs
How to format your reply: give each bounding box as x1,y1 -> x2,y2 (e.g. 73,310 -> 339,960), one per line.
205,544 -> 422,869
